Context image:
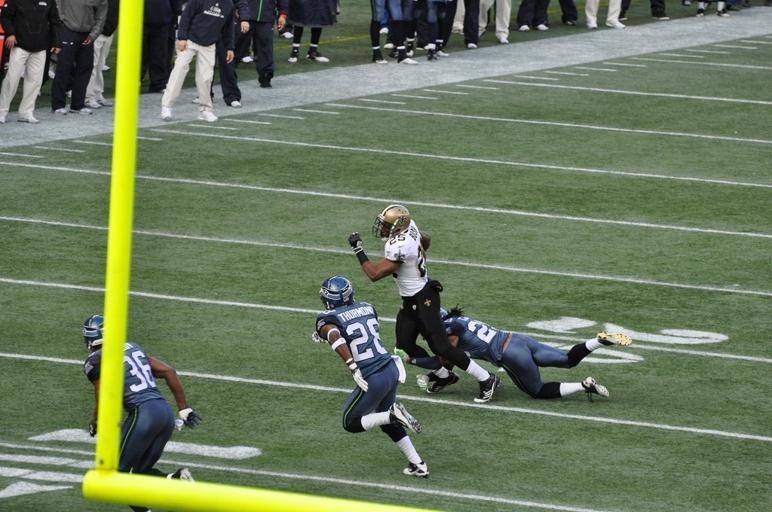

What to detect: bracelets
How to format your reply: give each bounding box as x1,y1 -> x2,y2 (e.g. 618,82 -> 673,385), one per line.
346,359 -> 358,373
356,250 -> 369,266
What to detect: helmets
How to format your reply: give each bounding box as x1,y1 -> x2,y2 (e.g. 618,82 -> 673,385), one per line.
319,275 -> 356,309
82,313 -> 105,350
381,204 -> 411,239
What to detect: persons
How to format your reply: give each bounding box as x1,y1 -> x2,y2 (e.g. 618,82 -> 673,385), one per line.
316,276 -> 430,477
394,308 -> 633,402
349,204 -> 501,404
81,313 -> 202,512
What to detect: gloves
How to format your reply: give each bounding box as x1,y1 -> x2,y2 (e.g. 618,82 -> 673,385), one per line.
347,230 -> 364,256
351,368 -> 370,392
176,405 -> 203,431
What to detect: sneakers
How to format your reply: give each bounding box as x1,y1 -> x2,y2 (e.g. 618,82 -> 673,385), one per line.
593,330 -> 633,347
424,370 -> 460,394
174,467 -> 195,482
389,401 -> 424,437
470,372 -> 501,403
582,377 -> 612,400
0,99 -> 112,124
401,460 -> 431,479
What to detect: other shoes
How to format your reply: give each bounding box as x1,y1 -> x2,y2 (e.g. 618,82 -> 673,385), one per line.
192,97 -> 200,104
619,13 -> 628,21
717,10 -> 730,17
535,24 -> 549,31
280,32 -> 294,39
260,82 -> 272,89
373,38 -> 449,65
102,65 -> 110,71
198,110 -> 218,123
231,100 -> 242,108
288,49 -> 300,63
696,8 -> 704,17
588,23 -> 598,29
565,20 -> 576,26
498,38 -> 509,43
606,21 -> 625,29
240,56 -> 253,64
467,43 -> 477,49
519,25 -> 530,31
652,11 -> 669,20
306,50 -> 329,63
160,108 -> 174,121
